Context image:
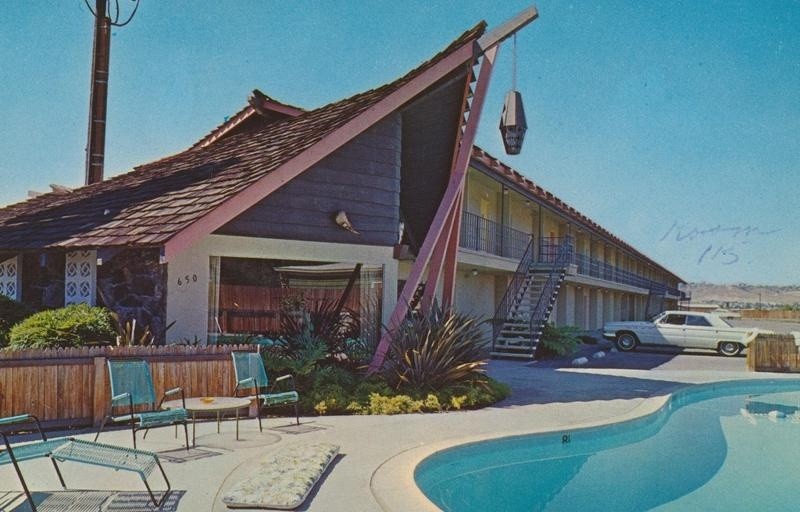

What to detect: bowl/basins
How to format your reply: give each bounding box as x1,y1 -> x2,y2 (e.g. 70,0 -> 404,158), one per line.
199,397 -> 215,404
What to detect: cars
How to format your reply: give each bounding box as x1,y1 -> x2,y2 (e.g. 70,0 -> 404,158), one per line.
714,308 -> 742,321
600,307 -> 775,355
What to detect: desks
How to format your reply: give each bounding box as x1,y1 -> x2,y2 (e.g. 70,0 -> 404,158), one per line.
162,396 -> 254,447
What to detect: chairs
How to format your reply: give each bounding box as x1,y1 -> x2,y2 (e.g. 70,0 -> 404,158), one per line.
228,348 -> 300,432
0,413 -> 174,511
92,356 -> 191,455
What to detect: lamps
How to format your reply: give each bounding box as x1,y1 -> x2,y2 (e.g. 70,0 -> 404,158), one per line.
499,30 -> 528,154
